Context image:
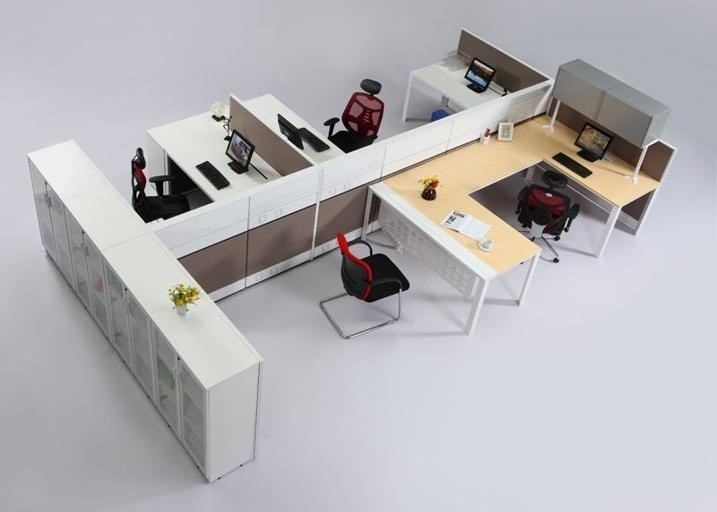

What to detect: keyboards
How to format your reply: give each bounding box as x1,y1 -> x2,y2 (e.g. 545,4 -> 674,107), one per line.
552,152 -> 593,178
196,161 -> 230,190
297,123 -> 329,152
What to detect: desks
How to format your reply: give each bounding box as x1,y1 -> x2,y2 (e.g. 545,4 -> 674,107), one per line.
400,54 -> 513,122
145,93 -> 350,203
359,113 -> 676,337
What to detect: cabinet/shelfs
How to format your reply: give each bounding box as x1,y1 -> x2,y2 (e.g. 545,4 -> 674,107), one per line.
21,135 -> 267,485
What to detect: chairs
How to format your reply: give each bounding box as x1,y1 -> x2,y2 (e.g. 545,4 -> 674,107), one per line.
319,231 -> 412,342
322,78 -> 385,150
130,144 -> 191,219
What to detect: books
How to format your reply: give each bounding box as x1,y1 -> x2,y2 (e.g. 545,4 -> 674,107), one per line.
440,208 -> 492,242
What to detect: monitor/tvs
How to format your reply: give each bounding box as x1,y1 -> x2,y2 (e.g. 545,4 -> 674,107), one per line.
225,129 -> 255,174
277,112 -> 305,150
463,57 -> 496,93
574,122 -> 614,162
